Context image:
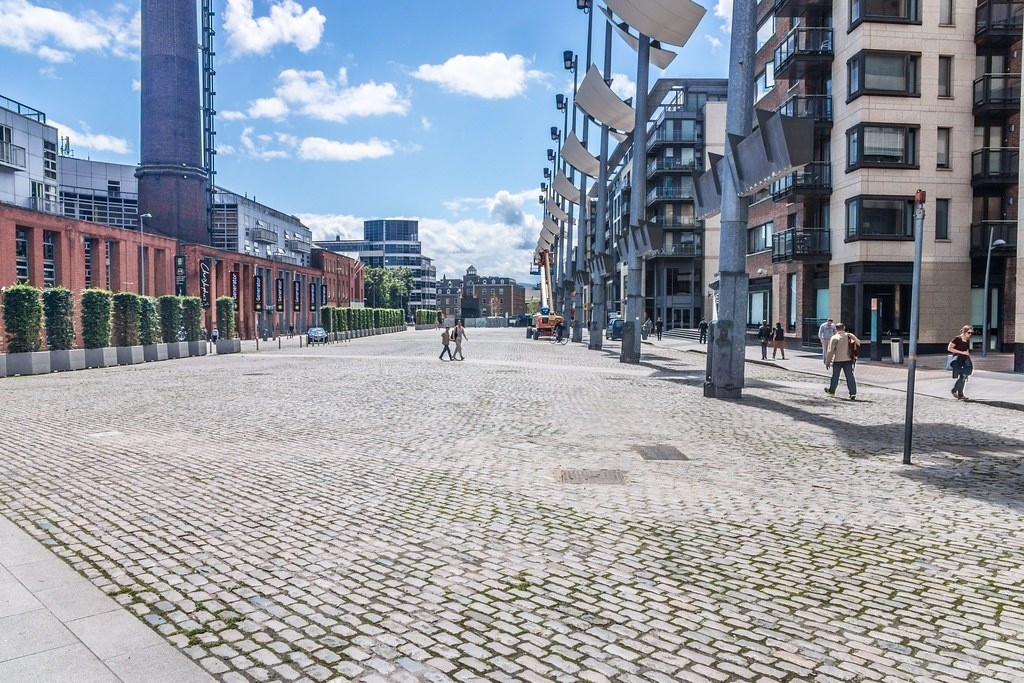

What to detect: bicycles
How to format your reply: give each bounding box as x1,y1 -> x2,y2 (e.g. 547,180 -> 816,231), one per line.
548,330 -> 569,345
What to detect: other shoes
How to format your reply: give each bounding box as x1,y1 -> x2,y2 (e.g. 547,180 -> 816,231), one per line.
958,396 -> 969,400
452,356 -> 456,359
849,395 -> 855,400
450,359 -> 454,361
762,358 -> 764,359
951,389 -> 958,398
824,388 -> 834,396
439,356 -> 443,360
461,357 -> 465,360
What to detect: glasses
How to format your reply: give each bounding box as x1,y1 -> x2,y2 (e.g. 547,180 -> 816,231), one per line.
967,331 -> 973,335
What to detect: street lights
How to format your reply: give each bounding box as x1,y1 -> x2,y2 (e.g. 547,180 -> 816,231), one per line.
550,126 -> 563,308
539,148 -> 557,312
571,0 -> 594,344
140,213 -> 153,295
555,93 -> 569,317
563,50 -> 578,340
373,276 -> 379,308
335,268 -> 342,306
982,226 -> 1007,356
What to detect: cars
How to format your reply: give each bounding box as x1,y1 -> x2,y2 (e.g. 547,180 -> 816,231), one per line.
305,327 -> 328,344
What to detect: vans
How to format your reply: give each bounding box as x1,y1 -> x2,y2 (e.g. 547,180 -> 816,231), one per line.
605,318 -> 624,341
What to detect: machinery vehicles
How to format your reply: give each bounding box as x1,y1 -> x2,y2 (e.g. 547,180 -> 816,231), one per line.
526,249 -> 567,340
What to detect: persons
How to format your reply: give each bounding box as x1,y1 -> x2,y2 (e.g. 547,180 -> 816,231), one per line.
819,319 -> 837,363
948,326 -> 973,400
554,322 -> 563,342
435,320 -> 440,331
646,318 -> 653,337
655,317 -> 664,341
758,320 -> 771,360
698,318 -> 708,344
290,323 -> 294,338
824,323 -> 860,400
211,328 -> 218,344
772,322 -> 785,359
439,320 -> 468,361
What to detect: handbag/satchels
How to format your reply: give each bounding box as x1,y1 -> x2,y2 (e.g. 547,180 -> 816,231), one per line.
946,354 -> 959,370
758,337 -> 767,344
451,327 -> 458,340
847,334 -> 859,360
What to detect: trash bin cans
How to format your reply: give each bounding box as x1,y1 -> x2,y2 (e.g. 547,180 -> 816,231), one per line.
891,338 -> 904,363
263,329 -> 268,341
527,327 -> 532,338
